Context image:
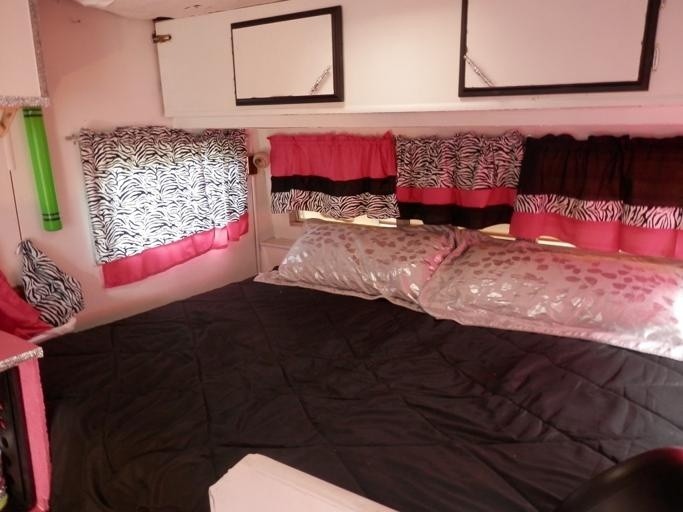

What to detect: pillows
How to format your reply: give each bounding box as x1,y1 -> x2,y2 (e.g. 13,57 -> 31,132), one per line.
252,219 -> 459,312
419,230 -> 683,363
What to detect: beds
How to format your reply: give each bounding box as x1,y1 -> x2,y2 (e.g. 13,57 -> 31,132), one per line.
0,265 -> 683,512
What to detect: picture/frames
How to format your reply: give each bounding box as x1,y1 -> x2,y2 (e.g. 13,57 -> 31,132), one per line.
458,0 -> 660,98
230,5 -> 345,106
